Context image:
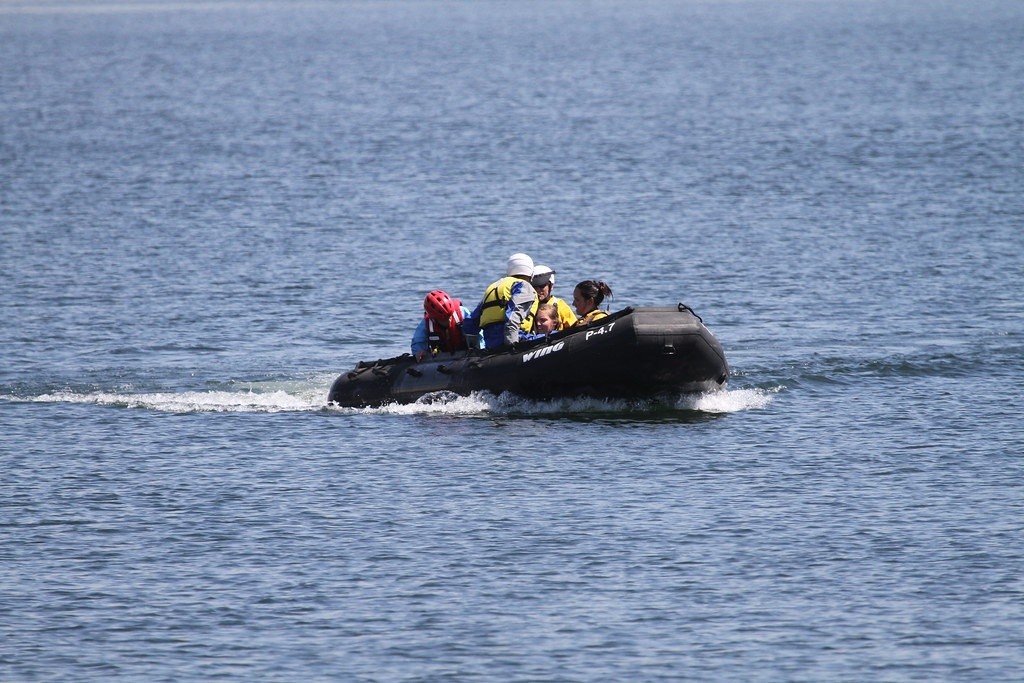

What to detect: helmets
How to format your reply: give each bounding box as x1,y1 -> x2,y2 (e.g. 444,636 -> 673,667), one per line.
506,253 -> 535,282
424,290 -> 454,318
531,265 -> 554,290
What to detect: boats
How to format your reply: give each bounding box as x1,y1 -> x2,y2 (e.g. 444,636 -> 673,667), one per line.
326,303 -> 729,407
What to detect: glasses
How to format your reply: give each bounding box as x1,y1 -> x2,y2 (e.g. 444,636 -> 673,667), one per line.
533,284 -> 546,288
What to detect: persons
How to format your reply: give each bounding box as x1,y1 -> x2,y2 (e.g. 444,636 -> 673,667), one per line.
411,290 -> 485,362
573,280 -> 613,327
536,303 -> 561,334
472,253 -> 559,352
530,265 -> 578,331
